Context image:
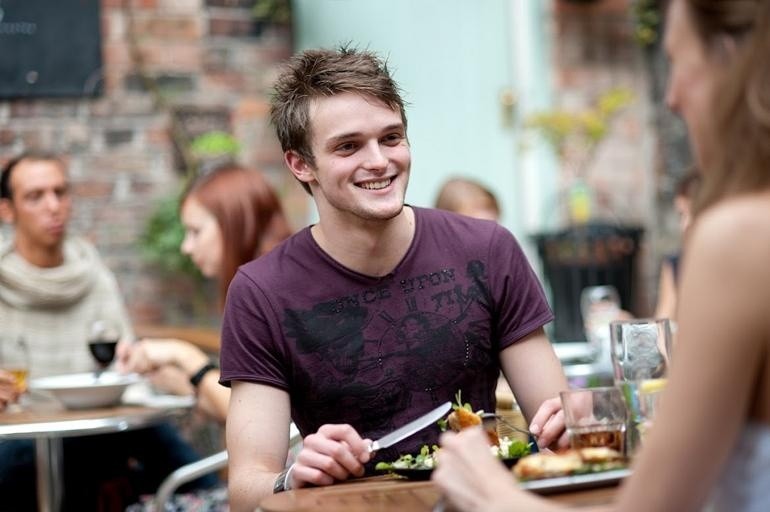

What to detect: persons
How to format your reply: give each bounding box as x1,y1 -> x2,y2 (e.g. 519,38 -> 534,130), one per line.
117,163 -> 297,512
0,150 -> 140,512
584,164 -> 704,342
432,0 -> 770,512
219,34 -> 591,511
429,175 -> 500,224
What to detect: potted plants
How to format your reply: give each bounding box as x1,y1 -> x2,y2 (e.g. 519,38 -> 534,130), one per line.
518,89 -> 633,221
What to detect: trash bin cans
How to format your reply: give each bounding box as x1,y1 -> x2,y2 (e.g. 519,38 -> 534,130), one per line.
529,225 -> 647,343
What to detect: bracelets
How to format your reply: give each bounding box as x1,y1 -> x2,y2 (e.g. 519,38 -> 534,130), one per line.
272,463 -> 295,492
189,363 -> 217,386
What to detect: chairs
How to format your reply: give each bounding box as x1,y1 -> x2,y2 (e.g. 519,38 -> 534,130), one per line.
154,423 -> 304,512
